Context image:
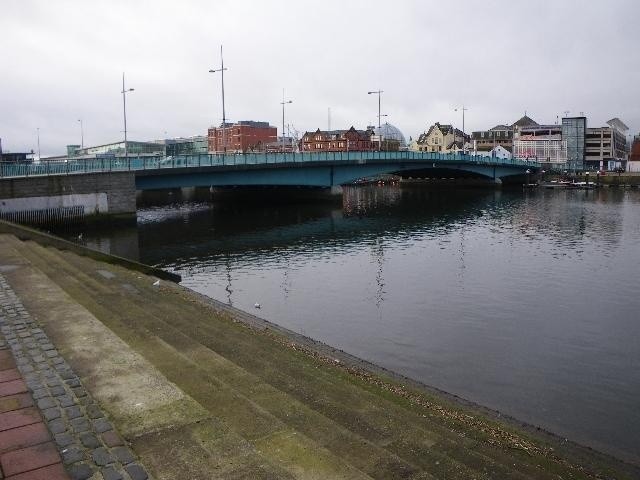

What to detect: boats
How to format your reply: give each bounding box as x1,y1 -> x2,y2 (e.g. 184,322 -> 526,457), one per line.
525,177 -> 599,189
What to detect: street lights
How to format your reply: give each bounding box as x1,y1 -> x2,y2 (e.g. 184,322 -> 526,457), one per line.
279,101 -> 294,151
76,118 -> 84,151
209,68 -> 229,127
454,103 -> 470,153
36,128 -> 40,160
367,90 -> 388,136
120,88 -> 136,140
